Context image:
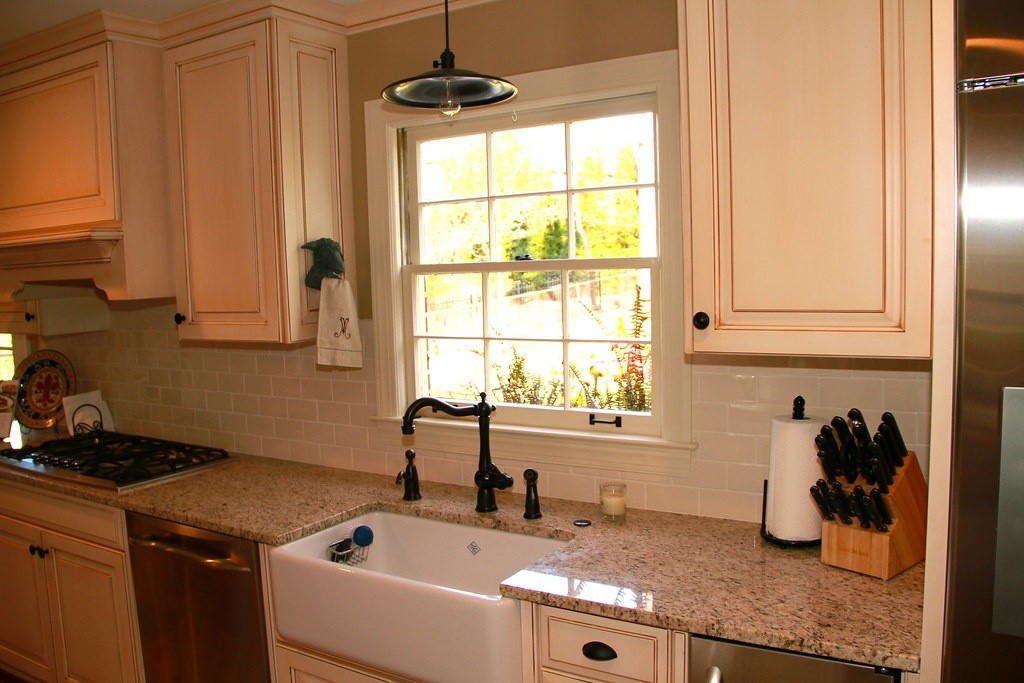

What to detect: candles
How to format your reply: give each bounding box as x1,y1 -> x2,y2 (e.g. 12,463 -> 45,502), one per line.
601,489 -> 626,516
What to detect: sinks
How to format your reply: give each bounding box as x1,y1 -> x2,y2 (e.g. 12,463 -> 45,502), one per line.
268,510 -> 569,683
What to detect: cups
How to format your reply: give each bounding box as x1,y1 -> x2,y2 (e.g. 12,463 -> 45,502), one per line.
600,481 -> 629,520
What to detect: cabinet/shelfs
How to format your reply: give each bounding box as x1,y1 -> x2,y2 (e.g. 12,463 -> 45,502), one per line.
0,296 -> 111,339
519,598 -> 689,683
675,0 -> 933,363
164,5 -> 357,349
0,9 -> 174,307
0,477 -> 147,683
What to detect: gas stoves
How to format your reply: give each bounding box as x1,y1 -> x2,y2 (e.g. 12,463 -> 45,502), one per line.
0,430 -> 241,497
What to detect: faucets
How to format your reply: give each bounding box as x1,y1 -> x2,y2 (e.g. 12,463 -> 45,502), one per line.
400,391 -> 515,512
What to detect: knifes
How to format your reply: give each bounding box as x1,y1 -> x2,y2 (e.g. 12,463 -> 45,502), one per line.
811,409 -> 908,533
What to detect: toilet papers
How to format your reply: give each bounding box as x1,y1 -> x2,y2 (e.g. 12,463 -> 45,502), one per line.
766,415 -> 834,543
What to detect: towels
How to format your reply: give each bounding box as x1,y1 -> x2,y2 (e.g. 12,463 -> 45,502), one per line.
315,276 -> 363,368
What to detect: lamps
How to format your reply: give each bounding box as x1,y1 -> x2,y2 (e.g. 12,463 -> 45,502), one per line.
379,0 -> 519,118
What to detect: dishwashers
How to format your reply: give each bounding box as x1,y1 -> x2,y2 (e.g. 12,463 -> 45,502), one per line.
126,510 -> 272,683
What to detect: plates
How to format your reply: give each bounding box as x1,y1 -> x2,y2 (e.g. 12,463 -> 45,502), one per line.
12,350 -> 77,429
